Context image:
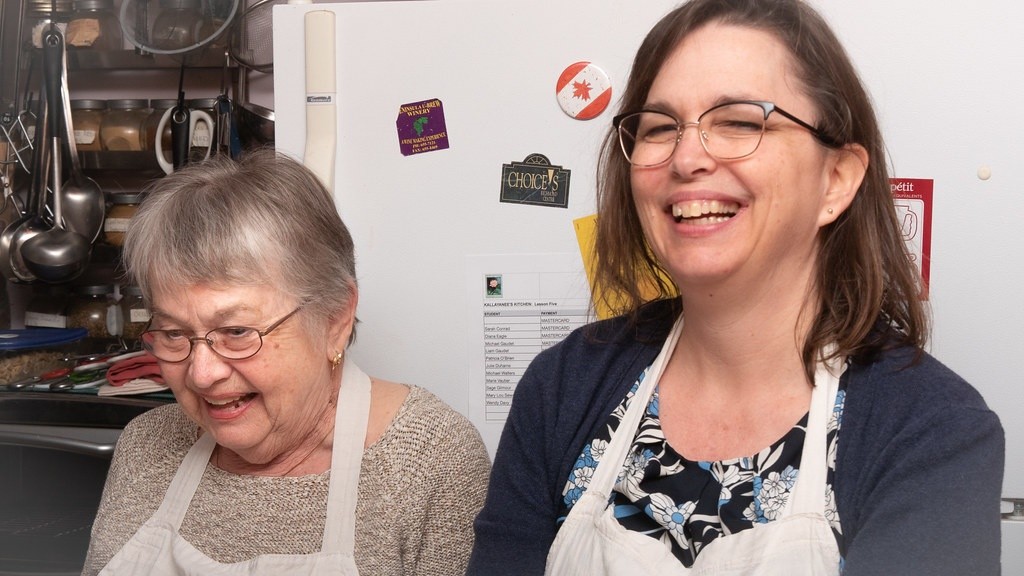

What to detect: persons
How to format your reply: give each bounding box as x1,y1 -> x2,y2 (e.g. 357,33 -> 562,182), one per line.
79,147 -> 492,576
467,0 -> 1006,576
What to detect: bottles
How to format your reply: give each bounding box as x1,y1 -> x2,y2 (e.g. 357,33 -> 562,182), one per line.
73,283 -> 151,343
21,0 -> 210,66
19,98 -> 221,151
103,190 -> 148,246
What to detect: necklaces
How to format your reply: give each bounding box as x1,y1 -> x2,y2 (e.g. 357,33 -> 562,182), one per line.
216,427 -> 334,475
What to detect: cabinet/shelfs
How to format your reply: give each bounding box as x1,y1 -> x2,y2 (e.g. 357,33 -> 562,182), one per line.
21,0 -> 255,172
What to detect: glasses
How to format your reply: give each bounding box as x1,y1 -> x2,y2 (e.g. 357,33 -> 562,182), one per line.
612,102 -> 846,168
140,284 -> 325,363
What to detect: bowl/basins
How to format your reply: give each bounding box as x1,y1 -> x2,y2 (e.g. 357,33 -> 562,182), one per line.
225,97 -> 274,146
